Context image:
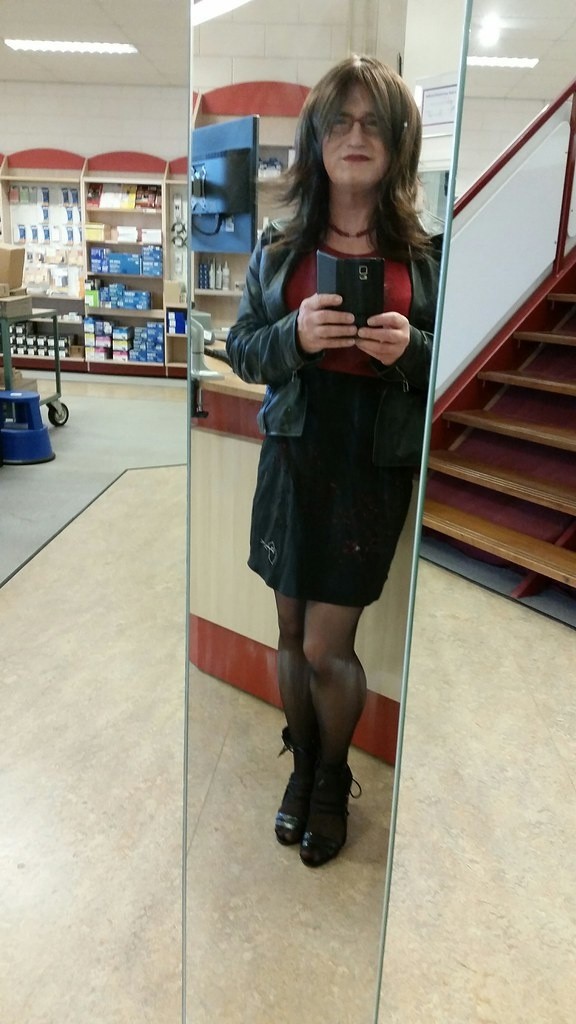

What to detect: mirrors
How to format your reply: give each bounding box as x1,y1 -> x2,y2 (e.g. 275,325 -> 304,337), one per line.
183,0 -> 473,1023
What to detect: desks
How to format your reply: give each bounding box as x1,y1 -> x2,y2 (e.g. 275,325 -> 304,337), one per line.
0,308 -> 69,426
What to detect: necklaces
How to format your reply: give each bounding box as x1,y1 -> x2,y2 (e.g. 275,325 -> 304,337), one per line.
328,224 -> 373,238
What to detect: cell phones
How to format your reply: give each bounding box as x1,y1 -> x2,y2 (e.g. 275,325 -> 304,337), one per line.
316,251 -> 385,345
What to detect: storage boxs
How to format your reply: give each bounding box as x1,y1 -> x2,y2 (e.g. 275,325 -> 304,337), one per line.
0,242 -> 32,318
89,246 -> 163,277
0,353 -> 38,392
99,283 -> 152,311
8,321 -> 83,357
84,318 -> 164,362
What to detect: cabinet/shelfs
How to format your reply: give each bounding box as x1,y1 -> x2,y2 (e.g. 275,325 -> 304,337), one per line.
192,81 -> 311,447
0,148 -> 187,378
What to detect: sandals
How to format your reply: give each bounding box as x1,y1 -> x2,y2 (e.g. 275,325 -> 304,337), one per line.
274,728 -> 315,848
299,764 -> 363,868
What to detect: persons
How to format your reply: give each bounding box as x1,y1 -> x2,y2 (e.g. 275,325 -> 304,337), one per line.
224,57 -> 447,868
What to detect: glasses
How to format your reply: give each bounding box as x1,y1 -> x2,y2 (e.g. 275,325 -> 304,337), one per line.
325,110 -> 385,139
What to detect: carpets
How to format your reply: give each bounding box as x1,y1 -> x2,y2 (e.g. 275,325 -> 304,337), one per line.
0,395 -> 188,588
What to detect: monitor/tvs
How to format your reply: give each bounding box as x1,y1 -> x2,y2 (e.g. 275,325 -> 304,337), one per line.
189,113 -> 258,255
